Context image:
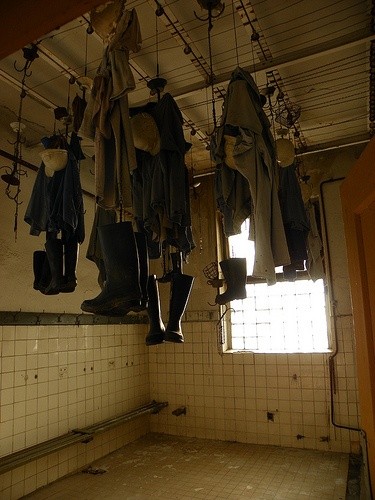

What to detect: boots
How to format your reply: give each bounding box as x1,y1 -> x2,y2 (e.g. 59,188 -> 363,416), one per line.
162,274 -> 195,343
81,221 -> 149,317
60,235 -> 79,293
33,250 -> 49,295
45,239 -> 63,295
158,252 -> 184,283
146,274 -> 166,346
215,258 -> 247,305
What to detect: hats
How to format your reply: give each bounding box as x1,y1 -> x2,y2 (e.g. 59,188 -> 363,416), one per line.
276,138 -> 295,167
224,134 -> 238,171
38,149 -> 67,177
130,112 -> 160,156
90,1 -> 124,38
